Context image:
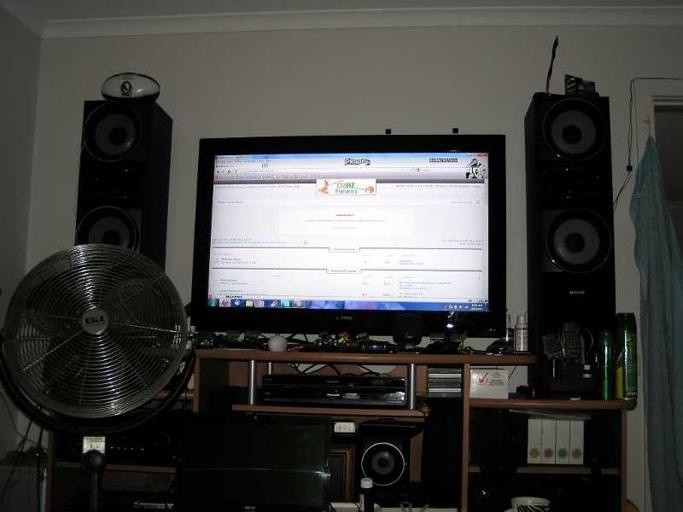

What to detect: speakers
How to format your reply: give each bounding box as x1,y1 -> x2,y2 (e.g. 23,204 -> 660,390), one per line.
344,414 -> 416,512
71,96 -> 172,274
522,90 -> 618,402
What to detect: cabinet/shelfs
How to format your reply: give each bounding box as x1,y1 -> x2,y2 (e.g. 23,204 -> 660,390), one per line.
45,347 -> 627,511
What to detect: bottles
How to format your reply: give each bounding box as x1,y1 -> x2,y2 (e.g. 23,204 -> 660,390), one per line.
502,313 -> 527,355
359,478 -> 374,512
581,364 -> 592,378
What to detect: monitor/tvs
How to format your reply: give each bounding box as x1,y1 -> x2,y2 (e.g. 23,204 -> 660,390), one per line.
190,132 -> 507,354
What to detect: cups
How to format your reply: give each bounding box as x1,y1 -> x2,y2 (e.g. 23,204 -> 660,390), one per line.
511,497 -> 549,512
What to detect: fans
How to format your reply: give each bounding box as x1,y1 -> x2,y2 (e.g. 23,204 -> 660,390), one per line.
3,242 -> 189,420
